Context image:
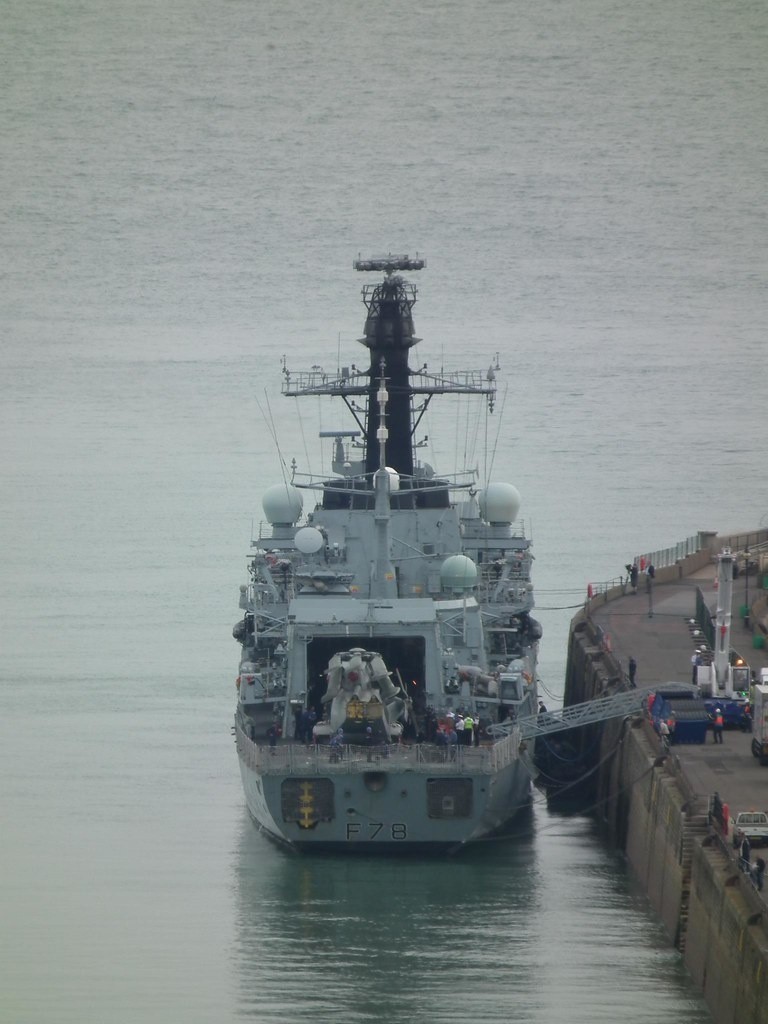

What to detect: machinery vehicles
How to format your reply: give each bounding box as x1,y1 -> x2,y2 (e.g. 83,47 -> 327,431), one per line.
691,548 -> 758,732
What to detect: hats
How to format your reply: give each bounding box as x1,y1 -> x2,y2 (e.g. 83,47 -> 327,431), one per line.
338,728 -> 343,734
458,715 -> 463,719
367,727 -> 371,732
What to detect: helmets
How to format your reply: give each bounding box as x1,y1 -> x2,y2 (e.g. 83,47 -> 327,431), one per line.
716,709 -> 720,713
745,698 -> 750,702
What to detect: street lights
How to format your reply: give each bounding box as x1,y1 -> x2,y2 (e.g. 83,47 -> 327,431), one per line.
741,544 -> 752,627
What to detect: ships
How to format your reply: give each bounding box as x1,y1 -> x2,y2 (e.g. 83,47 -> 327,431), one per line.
231,246 -> 548,860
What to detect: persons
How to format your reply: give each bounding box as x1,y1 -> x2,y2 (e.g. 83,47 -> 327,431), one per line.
739,832 -> 751,874
644,560 -> 655,593
294,705 -> 316,744
741,698 -> 752,733
266,724 -> 278,756
537,701 -> 547,728
630,564 -> 639,595
437,703 -> 480,747
628,656 -> 637,688
659,710 -> 676,745
756,853 -> 766,891
691,650 -> 701,684
707,708 -> 723,744
329,728 -> 344,764
365,726 -> 387,762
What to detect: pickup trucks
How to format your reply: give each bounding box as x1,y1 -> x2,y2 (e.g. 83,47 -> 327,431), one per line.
729,810 -> 768,848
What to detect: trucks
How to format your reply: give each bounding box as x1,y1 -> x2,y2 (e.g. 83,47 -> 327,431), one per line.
750,684 -> 768,767
